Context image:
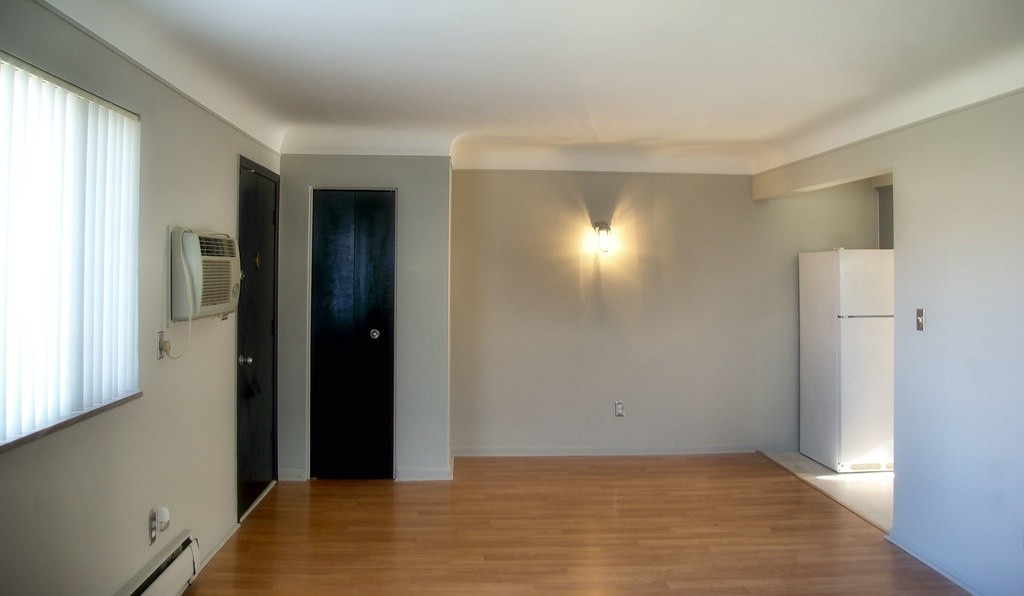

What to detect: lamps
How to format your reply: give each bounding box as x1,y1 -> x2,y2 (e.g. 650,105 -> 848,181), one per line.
594,224 -> 612,248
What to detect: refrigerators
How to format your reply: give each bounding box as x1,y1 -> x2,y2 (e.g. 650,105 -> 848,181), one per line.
798,247 -> 895,473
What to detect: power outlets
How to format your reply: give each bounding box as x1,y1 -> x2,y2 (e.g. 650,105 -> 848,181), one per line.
615,399 -> 625,417
157,331 -> 173,357
149,512 -> 157,545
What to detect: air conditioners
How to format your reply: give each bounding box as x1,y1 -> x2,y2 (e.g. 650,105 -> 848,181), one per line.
171,226 -> 240,321
